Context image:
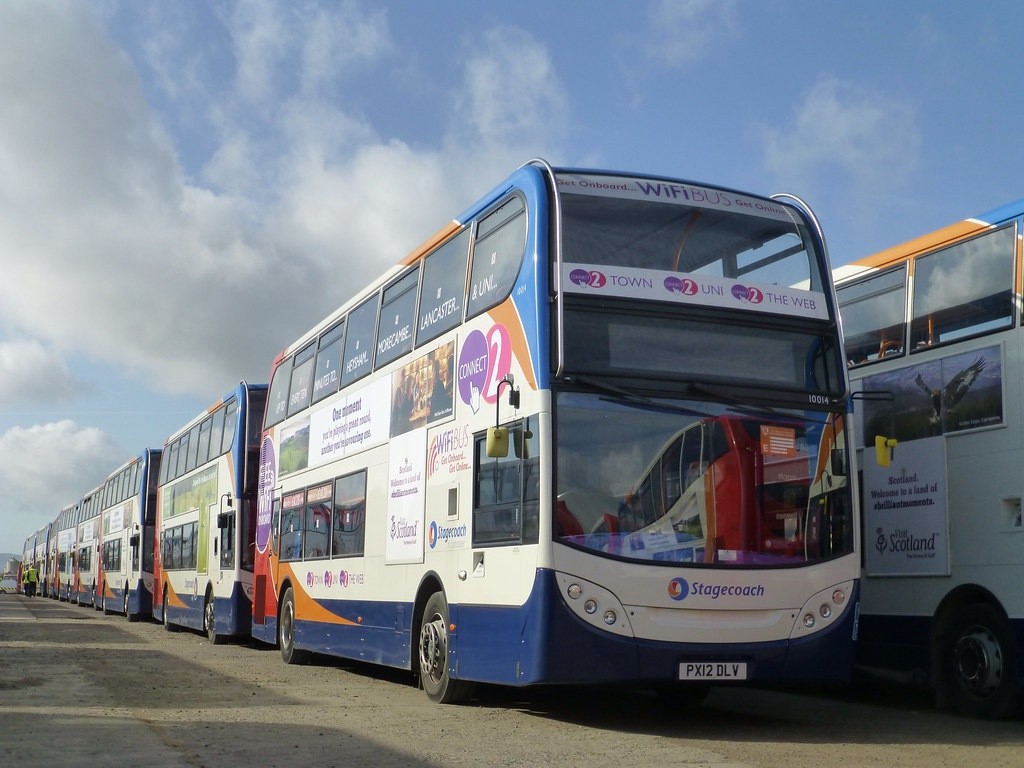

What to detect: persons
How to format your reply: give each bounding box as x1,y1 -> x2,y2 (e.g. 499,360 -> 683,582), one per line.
390,351 -> 456,442
25,565 -> 40,599
21,566 -> 29,598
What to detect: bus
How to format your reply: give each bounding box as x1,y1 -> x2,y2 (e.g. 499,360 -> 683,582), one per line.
152,379 -> 269,645
785,198 -> 1024,722
249,157 -> 863,704
20,447 -> 163,622
